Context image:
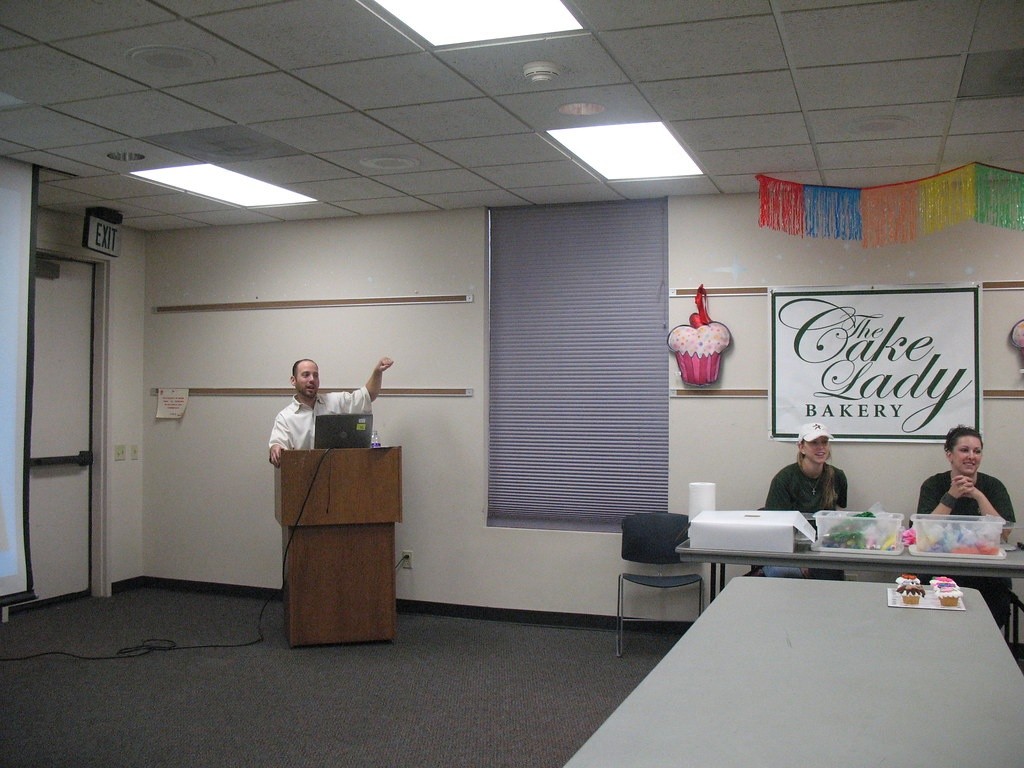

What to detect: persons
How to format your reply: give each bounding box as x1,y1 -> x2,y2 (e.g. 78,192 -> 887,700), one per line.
762,423 -> 847,580
912,423 -> 1016,631
268,356 -> 394,468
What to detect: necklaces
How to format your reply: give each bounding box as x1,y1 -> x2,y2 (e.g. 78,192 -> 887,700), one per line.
806,475 -> 822,495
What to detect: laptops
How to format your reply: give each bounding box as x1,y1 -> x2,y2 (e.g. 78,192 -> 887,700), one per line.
314,413 -> 373,449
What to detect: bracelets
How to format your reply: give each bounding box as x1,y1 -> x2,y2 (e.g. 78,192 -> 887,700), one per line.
940,492 -> 958,509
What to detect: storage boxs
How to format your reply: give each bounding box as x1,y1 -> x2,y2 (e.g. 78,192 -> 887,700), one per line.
687,510 -> 816,551
810,511 -> 905,553
910,512 -> 1006,553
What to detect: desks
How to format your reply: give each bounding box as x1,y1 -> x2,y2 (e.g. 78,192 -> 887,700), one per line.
676,538 -> 1024,663
562,575 -> 1024,768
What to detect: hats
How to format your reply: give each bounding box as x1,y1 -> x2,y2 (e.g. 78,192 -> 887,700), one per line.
797,423 -> 834,444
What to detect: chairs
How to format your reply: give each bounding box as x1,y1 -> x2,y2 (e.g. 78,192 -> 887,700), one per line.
615,510 -> 704,656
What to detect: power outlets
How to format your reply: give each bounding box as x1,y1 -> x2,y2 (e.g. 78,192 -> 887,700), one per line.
401,551 -> 414,570
